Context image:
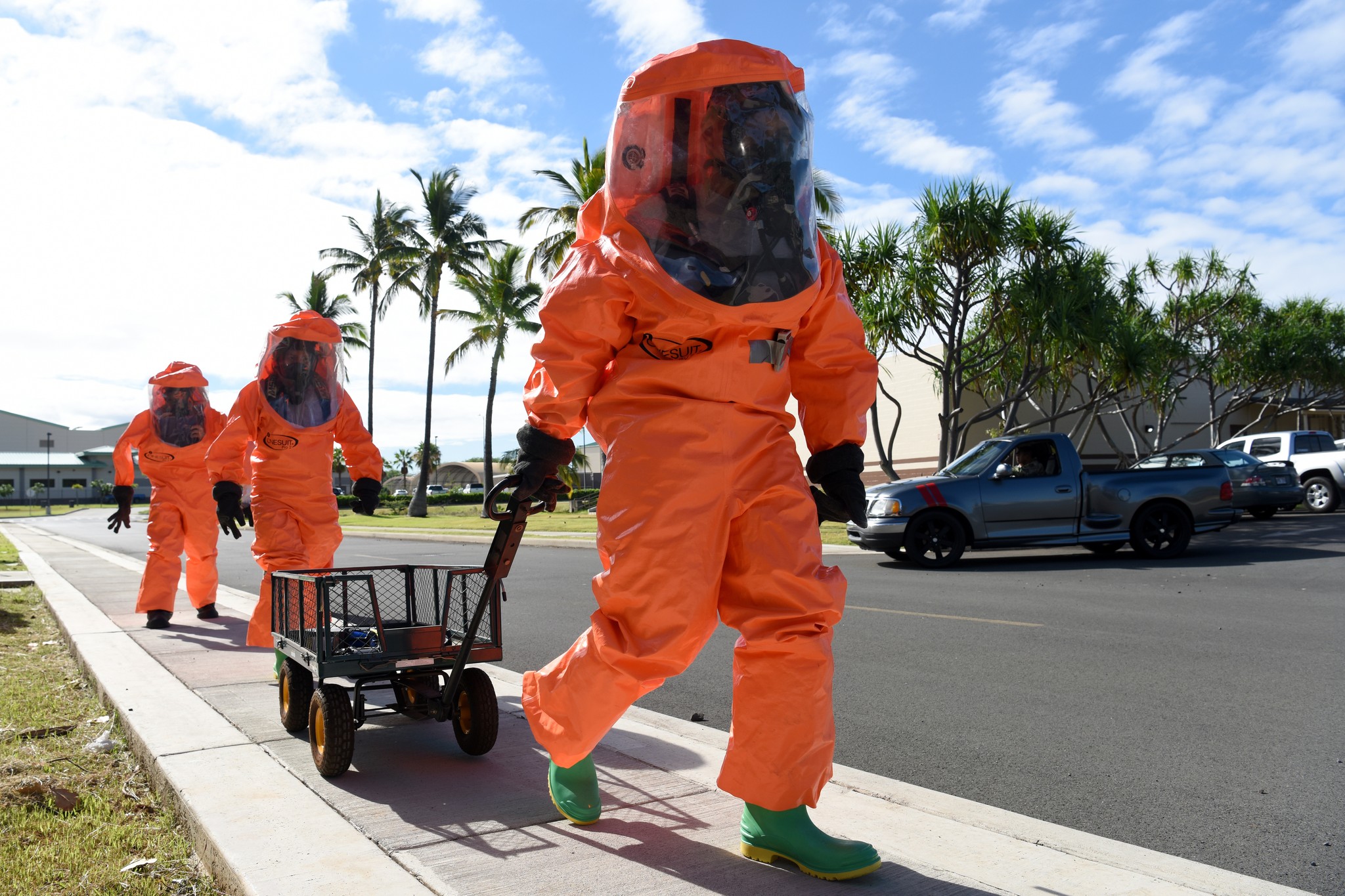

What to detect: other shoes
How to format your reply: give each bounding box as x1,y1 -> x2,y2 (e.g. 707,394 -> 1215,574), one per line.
273,647 -> 289,679
195,602 -> 219,619
146,609 -> 173,628
546,753 -> 602,825
740,799 -> 881,879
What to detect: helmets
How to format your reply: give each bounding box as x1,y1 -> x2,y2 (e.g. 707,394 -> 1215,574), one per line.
270,337 -> 319,406
699,81 -> 803,234
161,386 -> 192,418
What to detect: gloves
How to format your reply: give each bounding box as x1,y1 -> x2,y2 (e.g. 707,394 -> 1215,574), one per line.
108,485 -> 135,533
349,477 -> 382,515
504,424 -> 578,514
806,441 -> 869,528
212,480 -> 246,539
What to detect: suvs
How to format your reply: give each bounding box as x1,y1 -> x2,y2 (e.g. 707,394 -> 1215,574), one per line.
463,483 -> 485,494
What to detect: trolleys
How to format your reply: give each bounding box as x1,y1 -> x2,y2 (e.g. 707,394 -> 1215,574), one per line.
268,476 -> 546,777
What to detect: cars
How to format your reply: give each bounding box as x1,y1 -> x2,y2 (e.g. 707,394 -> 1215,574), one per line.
1126,449 -> 1310,520
392,490 -> 409,496
332,487 -> 344,496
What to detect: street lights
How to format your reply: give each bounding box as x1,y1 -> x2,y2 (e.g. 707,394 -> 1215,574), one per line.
582,426 -> 586,490
435,436 -> 439,484
46,432 -> 53,514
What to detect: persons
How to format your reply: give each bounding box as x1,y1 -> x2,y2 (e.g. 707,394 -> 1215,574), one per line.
507,38 -> 883,881
1010,442 -> 1044,478
106,361 -> 228,630
204,309 -> 383,678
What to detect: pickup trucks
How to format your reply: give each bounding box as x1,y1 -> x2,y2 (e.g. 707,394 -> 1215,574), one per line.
426,485 -> 449,495
845,433 -> 1243,567
1212,430 -> 1345,512
